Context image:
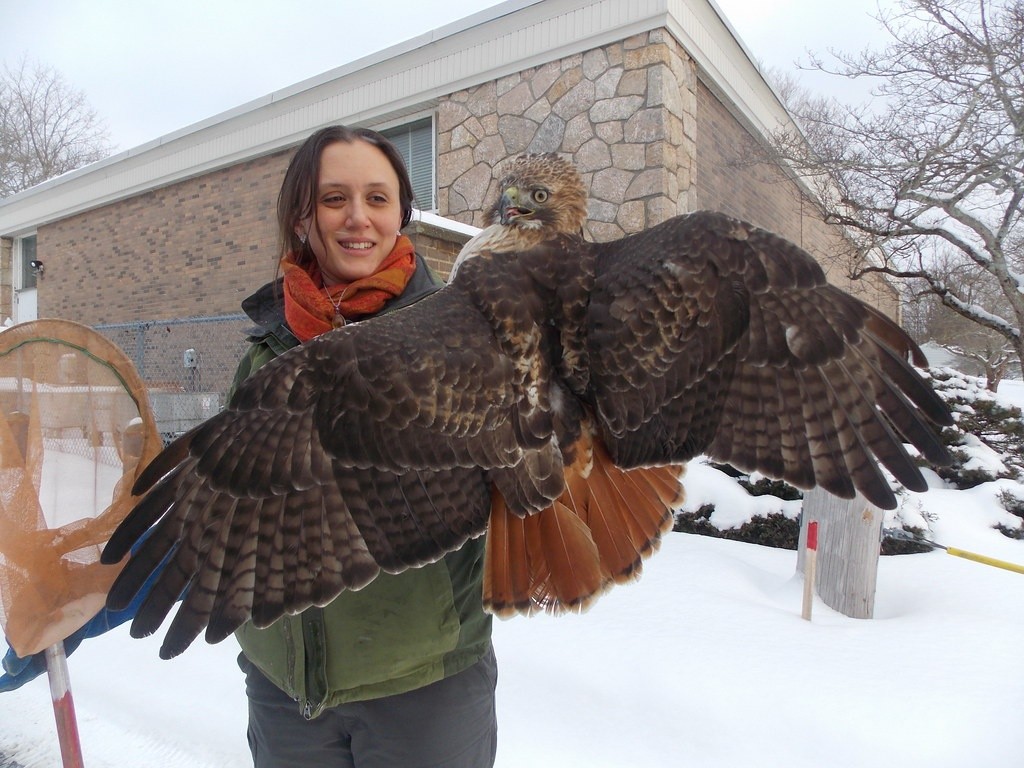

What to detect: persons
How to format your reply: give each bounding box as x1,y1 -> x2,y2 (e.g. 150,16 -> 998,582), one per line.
221,126 -> 500,768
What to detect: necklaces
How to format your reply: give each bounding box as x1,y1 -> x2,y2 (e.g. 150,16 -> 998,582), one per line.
323,279 -> 347,328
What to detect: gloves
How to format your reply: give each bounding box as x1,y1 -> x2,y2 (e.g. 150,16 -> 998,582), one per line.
0,600 -> 88,692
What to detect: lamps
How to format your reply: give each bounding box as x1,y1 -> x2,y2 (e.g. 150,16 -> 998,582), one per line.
29,260 -> 43,268
